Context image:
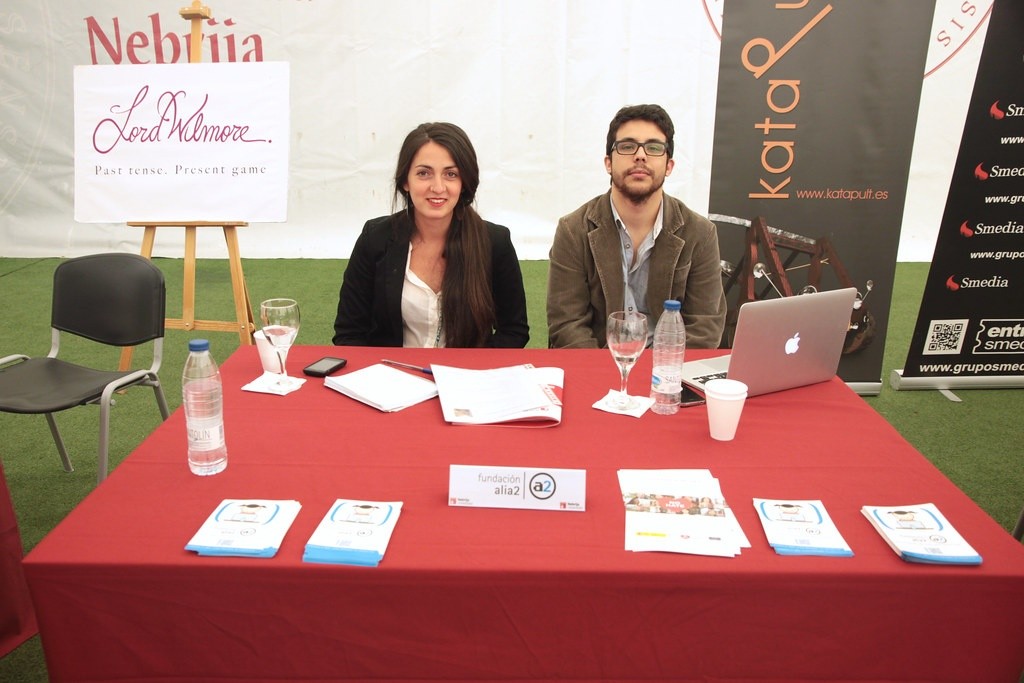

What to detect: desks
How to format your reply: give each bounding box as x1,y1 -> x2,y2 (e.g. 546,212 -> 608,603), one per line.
19,343 -> 1024,682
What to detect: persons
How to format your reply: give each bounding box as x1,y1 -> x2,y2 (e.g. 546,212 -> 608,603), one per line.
546,104 -> 727,348
331,123 -> 531,348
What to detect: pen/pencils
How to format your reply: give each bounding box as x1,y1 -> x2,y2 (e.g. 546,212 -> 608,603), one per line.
382,359 -> 432,374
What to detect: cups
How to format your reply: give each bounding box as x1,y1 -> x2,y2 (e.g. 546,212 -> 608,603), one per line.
703,379 -> 748,442
252,329 -> 290,376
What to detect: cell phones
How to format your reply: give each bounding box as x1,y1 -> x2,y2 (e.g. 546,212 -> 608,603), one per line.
303,357 -> 347,377
679,383 -> 706,406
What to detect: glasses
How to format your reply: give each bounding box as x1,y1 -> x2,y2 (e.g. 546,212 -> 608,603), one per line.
610,141 -> 670,157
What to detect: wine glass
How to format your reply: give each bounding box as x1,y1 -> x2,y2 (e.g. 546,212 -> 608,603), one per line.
606,311 -> 649,410
260,298 -> 301,394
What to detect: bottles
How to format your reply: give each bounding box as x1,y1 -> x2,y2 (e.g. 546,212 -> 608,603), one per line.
650,299 -> 686,415
183,338 -> 228,476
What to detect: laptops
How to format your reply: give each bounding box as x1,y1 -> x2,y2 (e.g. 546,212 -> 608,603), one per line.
652,288 -> 857,398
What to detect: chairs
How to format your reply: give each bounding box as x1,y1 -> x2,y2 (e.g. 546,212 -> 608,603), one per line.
1,253 -> 170,487
